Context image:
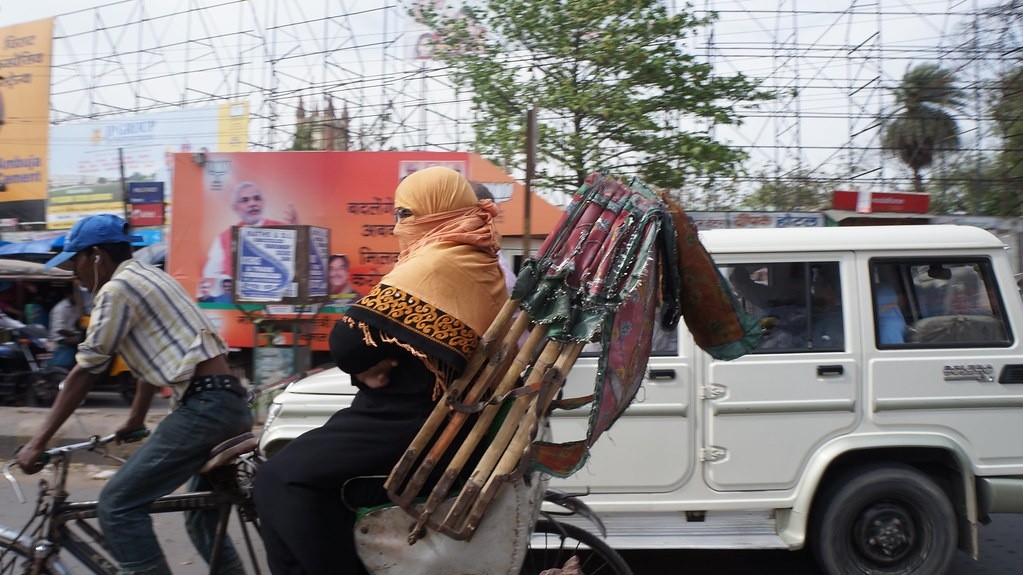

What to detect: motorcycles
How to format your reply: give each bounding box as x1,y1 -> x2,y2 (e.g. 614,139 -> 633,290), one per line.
1,260 -> 136,409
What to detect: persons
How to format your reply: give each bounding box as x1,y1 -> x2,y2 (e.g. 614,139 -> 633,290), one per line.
195,182 -> 286,296
327,256 -> 363,304
255,166 -> 520,574
468,180 -> 528,349
215,280 -> 233,302
727,263 -> 996,353
197,281 -> 216,302
15,214 -> 255,575
0,281 -> 85,376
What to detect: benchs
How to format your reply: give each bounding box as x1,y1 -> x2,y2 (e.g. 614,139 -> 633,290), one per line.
910,314 -> 1003,343
352,411 -> 554,574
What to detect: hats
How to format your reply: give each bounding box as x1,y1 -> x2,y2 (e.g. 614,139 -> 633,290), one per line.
45,213 -> 134,270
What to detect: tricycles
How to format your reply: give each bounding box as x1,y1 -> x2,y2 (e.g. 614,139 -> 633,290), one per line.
0,172 -> 763,574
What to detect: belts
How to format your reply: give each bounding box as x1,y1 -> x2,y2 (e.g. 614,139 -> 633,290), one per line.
182,376 -> 244,397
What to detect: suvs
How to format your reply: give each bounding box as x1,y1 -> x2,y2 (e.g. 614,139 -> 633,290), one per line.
256,225 -> 1023,574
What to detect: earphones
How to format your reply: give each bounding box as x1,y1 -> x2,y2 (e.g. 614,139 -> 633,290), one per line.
94,255 -> 100,263
